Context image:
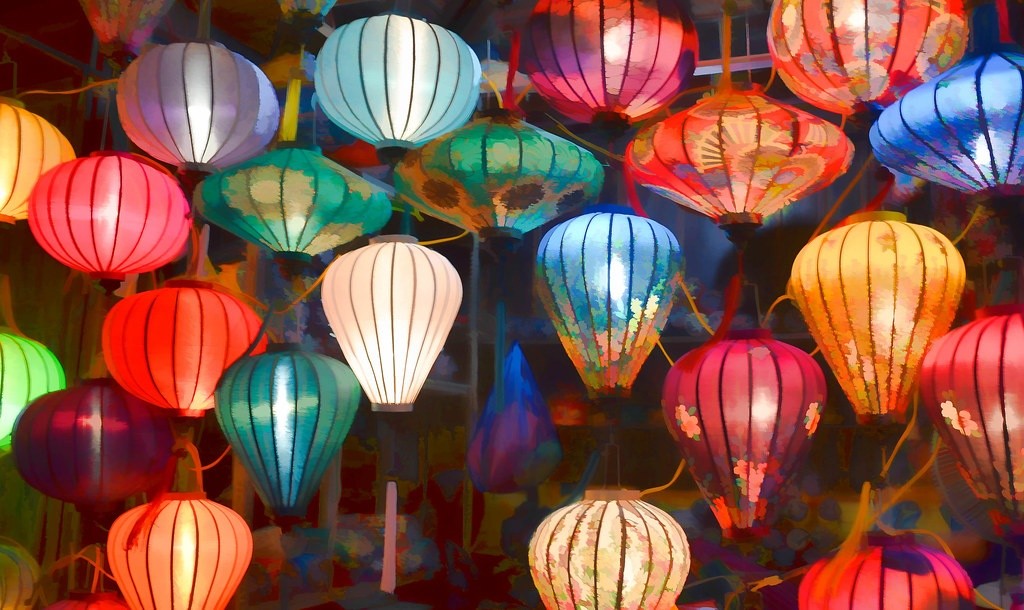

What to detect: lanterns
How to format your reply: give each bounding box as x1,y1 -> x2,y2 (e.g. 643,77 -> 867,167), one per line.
0,0 -> 1024,610
